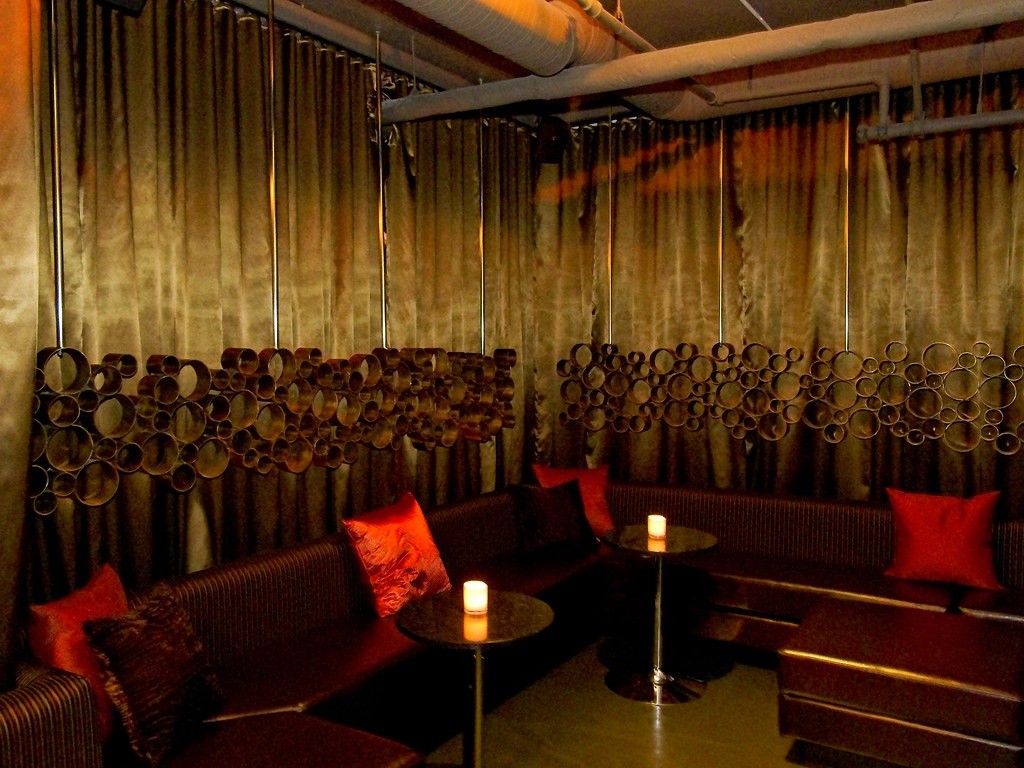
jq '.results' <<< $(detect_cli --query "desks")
[396,591,555,768]
[601,526,721,704]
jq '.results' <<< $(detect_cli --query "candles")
[464,615,488,642]
[647,537,665,551]
[648,515,666,537]
[463,581,487,614]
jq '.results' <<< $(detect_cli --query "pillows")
[31,564,130,741]
[515,480,599,559]
[885,486,1001,592]
[340,492,451,619]
[82,580,226,764]
[532,464,614,537]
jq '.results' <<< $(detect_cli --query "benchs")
[777,597,1024,768]
[0,484,599,768]
[600,483,1023,672]
